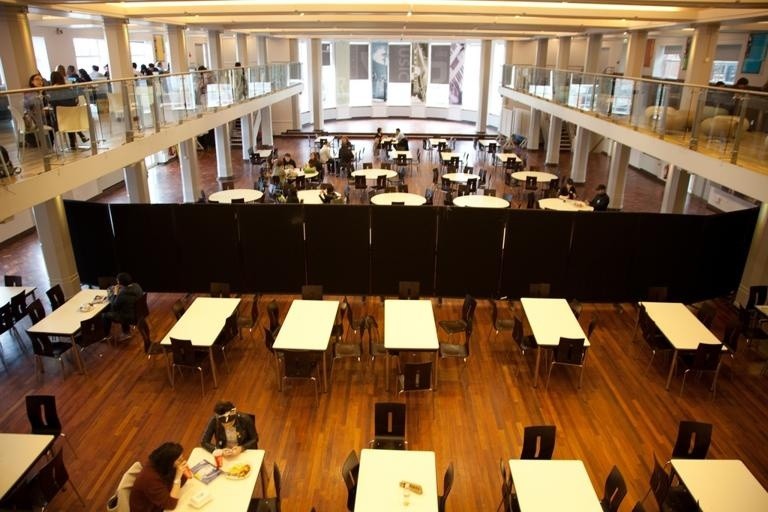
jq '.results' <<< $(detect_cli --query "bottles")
[397,481,422,494]
[402,492,410,506]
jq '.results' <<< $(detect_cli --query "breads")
[400,480,423,494]
[228,464,250,476]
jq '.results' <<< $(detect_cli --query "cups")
[212,449,224,468]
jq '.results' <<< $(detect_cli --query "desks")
[353,447,438,512]
[0,429,55,502]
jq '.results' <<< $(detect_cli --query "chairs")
[0,61,179,185]
[263,286,356,410]
[0,272,39,357]
[25,276,134,382]
[437,461,455,511]
[145,281,257,399]
[367,402,409,449]
[498,422,767,512]
[109,410,281,510]
[625,288,740,403]
[341,449,359,508]
[194,132,596,211]
[367,284,476,411]
[489,280,600,402]
[26,396,78,495]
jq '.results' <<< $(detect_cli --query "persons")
[372,128,403,155]
[21,58,245,153]
[128,442,192,512]
[557,178,575,199]
[266,134,352,205]
[714,78,748,126]
[585,184,608,211]
[199,401,258,457]
[98,272,144,343]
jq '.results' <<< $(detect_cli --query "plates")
[79,303,95,312]
[227,462,251,479]
